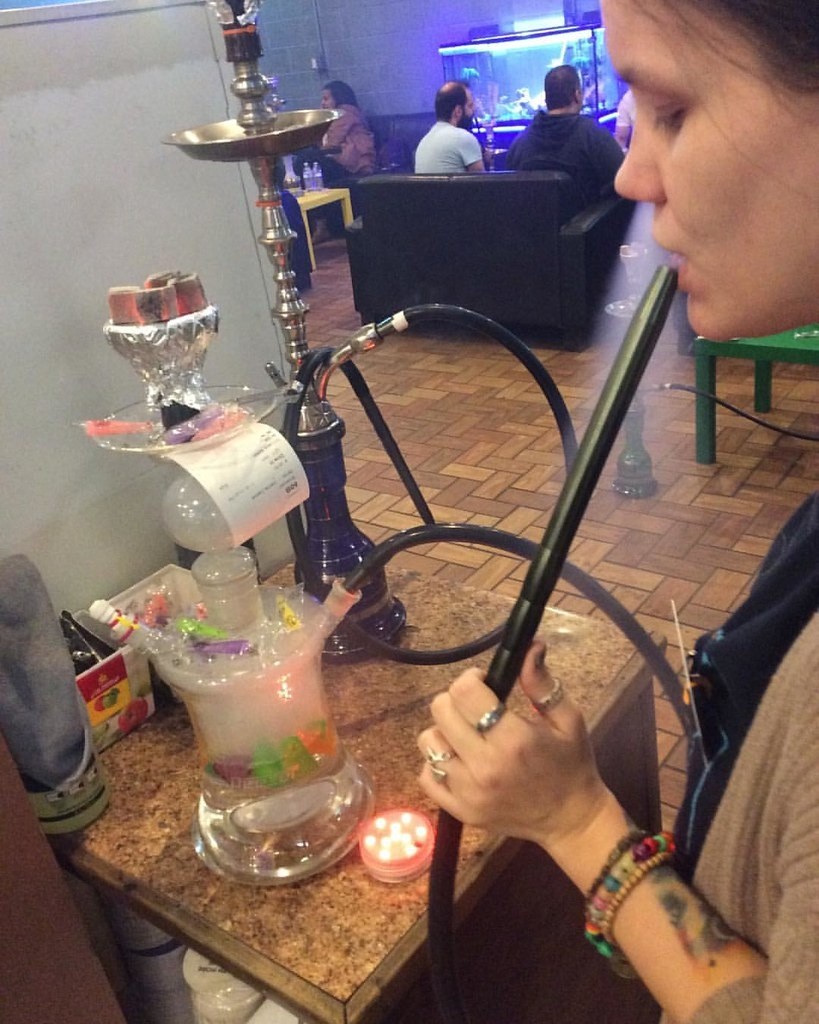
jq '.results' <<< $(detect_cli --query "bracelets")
[578,825,683,957]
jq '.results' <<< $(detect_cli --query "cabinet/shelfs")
[46,553,676,1024]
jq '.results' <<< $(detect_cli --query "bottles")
[313,162,324,191]
[303,162,314,192]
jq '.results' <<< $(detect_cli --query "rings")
[423,746,457,784]
[475,703,507,732]
[529,678,565,712]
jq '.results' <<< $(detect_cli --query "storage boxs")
[437,23,624,132]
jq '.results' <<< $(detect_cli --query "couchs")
[344,170,643,349]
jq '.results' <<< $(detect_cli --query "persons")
[614,82,641,152]
[276,160,314,296]
[505,66,626,202]
[295,80,377,244]
[416,0,819,1024]
[416,82,487,174]
